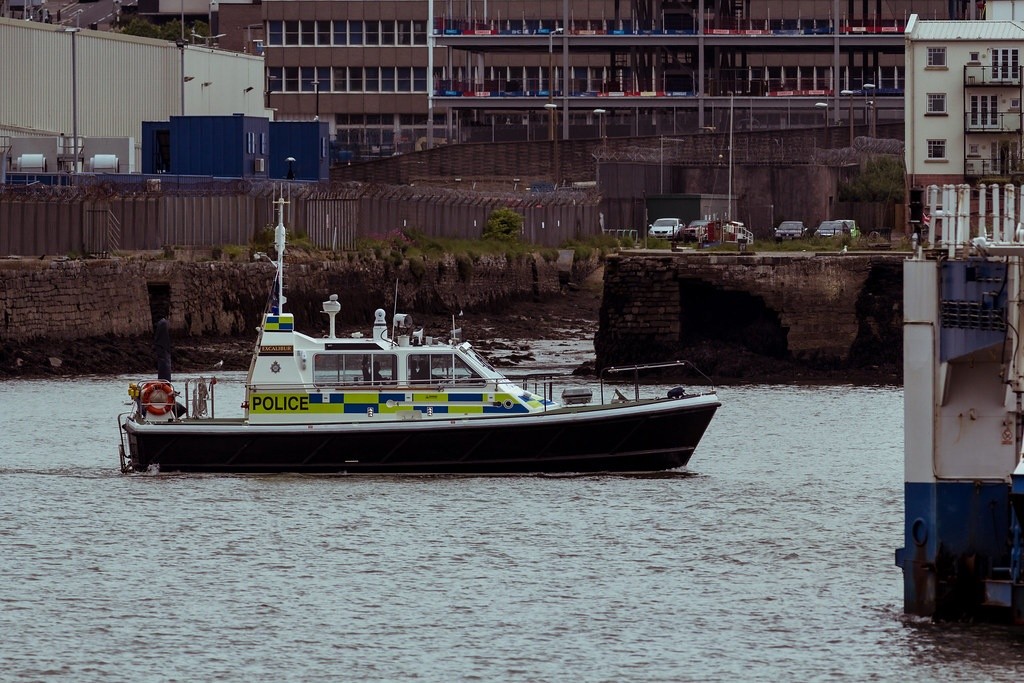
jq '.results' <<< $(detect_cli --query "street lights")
[548,27,565,98]
[842,89,855,162]
[815,102,829,126]
[864,83,876,125]
[65,26,83,173]
[594,108,607,136]
[544,104,558,141]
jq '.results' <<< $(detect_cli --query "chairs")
[967,164,975,175]
[361,357,370,385]
[411,360,417,384]
[982,163,989,175]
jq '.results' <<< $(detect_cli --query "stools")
[968,76,975,85]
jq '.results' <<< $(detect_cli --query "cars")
[835,219,861,238]
[815,221,852,239]
[647,217,687,241]
[773,220,809,243]
[683,219,718,243]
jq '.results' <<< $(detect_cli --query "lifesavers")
[141,383,174,416]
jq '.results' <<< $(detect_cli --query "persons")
[411,360,428,384]
[374,361,387,385]
[841,222,851,247]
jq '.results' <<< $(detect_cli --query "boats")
[129,181,722,476]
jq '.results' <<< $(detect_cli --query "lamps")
[244,86,253,92]
[184,76,195,82]
[202,82,212,86]
